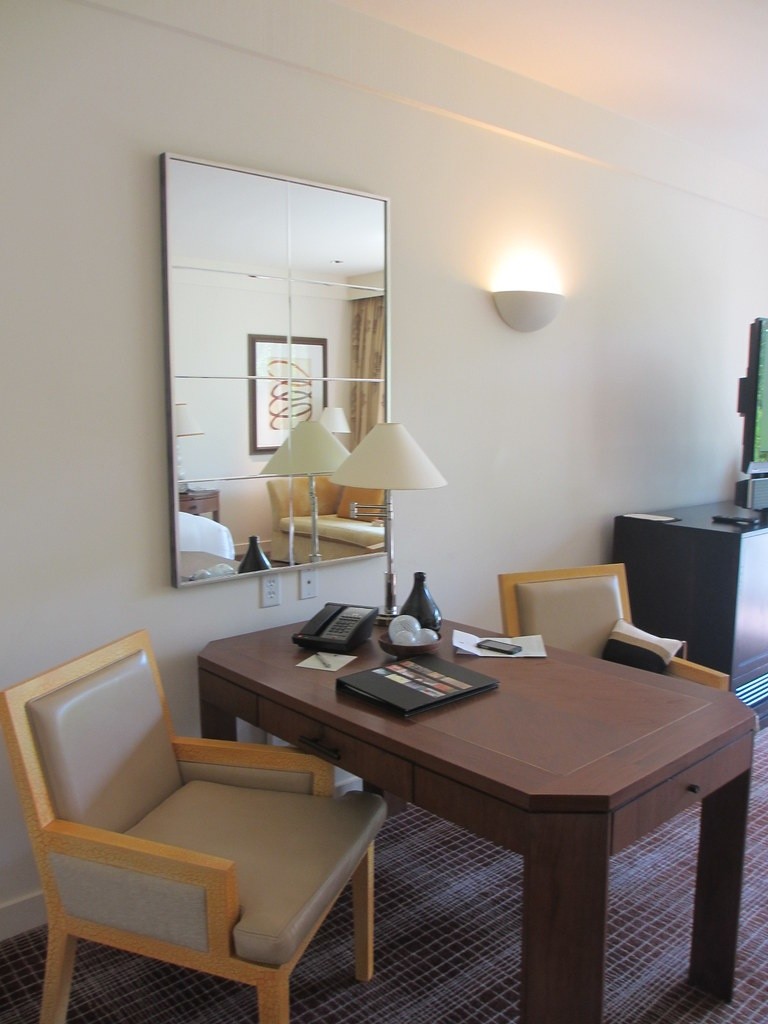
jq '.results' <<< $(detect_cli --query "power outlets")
[261,574,282,607]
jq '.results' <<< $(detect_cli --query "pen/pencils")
[310,649,333,667]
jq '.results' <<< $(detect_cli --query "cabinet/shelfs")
[179,490,221,522]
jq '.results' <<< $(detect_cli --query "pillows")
[601,619,684,673]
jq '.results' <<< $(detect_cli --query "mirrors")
[161,152,389,588]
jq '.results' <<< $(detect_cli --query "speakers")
[734,478,768,510]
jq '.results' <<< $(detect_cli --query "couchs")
[265,477,385,563]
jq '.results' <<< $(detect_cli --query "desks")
[180,551,241,581]
[198,618,758,1024]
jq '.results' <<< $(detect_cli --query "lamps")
[329,423,448,625]
[492,290,564,333]
[319,407,351,433]
[176,403,205,493]
[259,420,351,562]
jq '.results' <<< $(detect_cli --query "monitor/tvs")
[741,318,768,474]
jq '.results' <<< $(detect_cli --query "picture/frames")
[249,334,328,454]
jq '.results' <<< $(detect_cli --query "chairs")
[0,629,389,1024]
[497,564,730,691]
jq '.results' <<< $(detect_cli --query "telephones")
[292,601,380,655]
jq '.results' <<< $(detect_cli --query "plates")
[378,628,443,657]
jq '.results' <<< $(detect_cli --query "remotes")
[712,515,760,525]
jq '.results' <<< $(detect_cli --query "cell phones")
[477,639,522,655]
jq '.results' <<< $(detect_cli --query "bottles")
[397,571,442,633]
[237,535,272,575]
